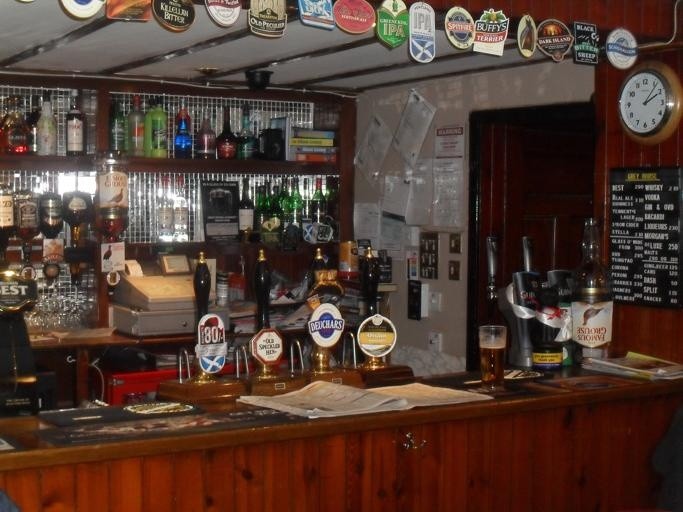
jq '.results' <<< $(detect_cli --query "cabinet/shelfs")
[1,69,356,407]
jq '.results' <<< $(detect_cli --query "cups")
[479,324,505,389]
[23,292,96,333]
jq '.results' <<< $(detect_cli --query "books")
[234,379,496,420]
[580,350,683,380]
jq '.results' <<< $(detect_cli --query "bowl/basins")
[245,70,273,90]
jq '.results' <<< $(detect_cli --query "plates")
[502,369,544,380]
[124,401,193,416]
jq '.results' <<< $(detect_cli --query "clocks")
[616,61,683,146]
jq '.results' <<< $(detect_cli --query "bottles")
[215,271,229,306]
[95,152,129,243]
[64,88,85,157]
[192,252,211,325]
[108,94,270,159]
[531,285,564,369]
[156,176,173,242]
[311,247,325,284]
[12,190,40,266]
[25,121,36,154]
[573,218,613,364]
[39,193,62,264]
[0,184,12,267]
[36,95,57,157]
[0,95,28,155]
[173,176,188,243]
[254,249,270,322]
[238,175,337,249]
[360,246,375,294]
[62,191,91,251]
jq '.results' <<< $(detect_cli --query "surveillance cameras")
[247,71,271,92]
